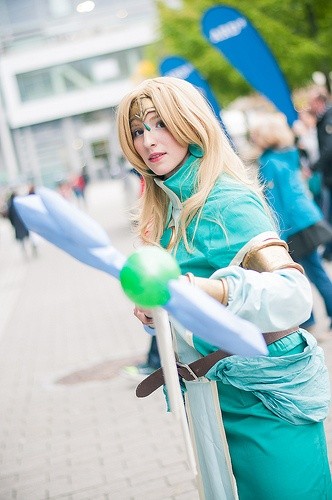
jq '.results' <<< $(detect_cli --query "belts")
[136,325,299,398]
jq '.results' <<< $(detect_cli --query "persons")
[59,165,90,205]
[249,111,331,343]
[6,181,39,262]
[116,77,332,500]
[293,85,332,265]
